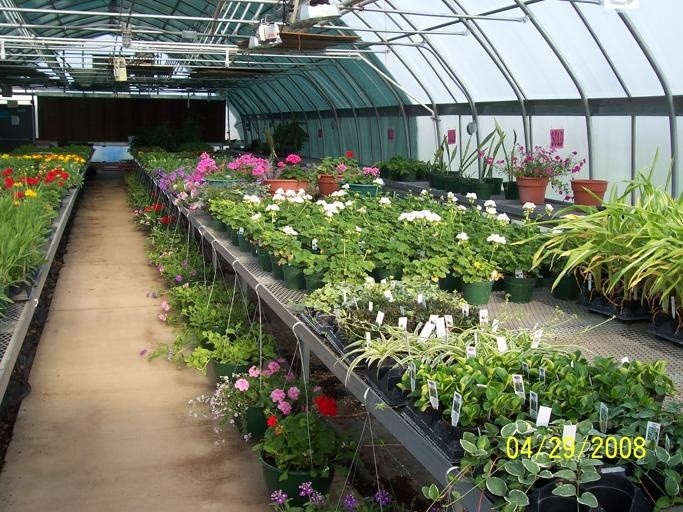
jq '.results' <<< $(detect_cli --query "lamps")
[122,23,131,48]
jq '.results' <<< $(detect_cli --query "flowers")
[122,138,683,512]
[1,140,94,323]
